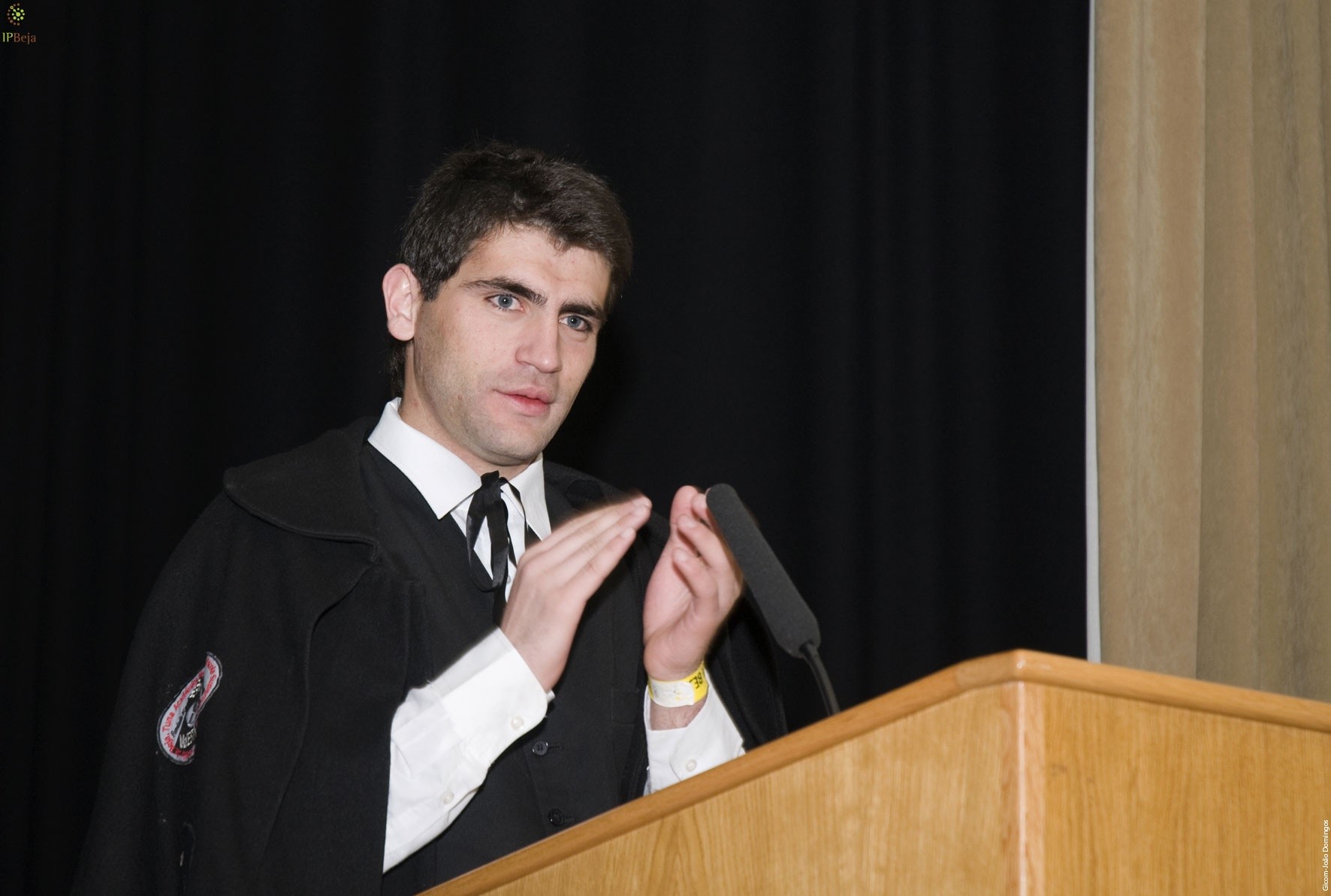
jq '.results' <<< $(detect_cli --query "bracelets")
[647,659,709,708]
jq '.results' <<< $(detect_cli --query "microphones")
[705,482,839,715]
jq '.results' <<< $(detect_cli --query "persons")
[81,142,788,896]
